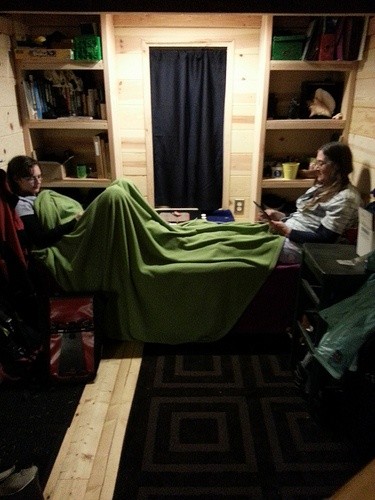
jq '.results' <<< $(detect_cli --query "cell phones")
[253,201,271,221]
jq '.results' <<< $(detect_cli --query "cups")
[282,162,300,179]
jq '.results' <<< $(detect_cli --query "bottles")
[271,166,283,178]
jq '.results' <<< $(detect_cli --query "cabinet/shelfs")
[9,6,118,190]
[251,16,370,224]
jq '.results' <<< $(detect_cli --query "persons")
[179,142,359,266]
[7,155,141,268]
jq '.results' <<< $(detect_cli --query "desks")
[300,241,364,333]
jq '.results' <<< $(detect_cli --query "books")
[24,74,106,119]
[94,135,112,179]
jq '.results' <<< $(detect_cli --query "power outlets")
[233,199,244,214]
